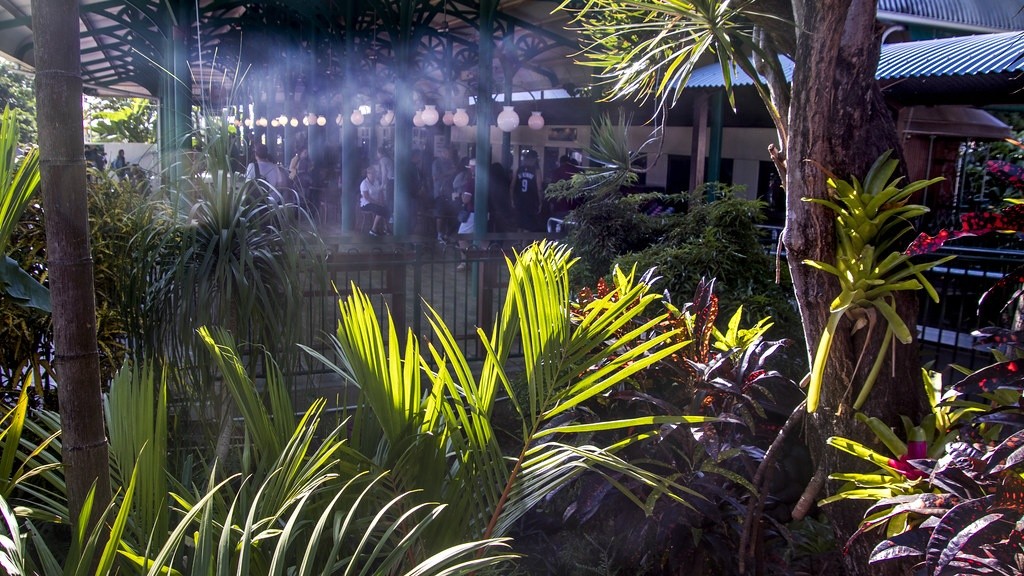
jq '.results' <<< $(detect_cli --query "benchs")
[448,232,570,241]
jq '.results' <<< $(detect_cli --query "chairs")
[316,188,338,226]
[548,218,576,233]
[356,192,393,236]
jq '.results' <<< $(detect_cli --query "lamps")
[227,81,545,132]
[562,83,580,98]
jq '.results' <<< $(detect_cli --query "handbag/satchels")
[245,162,269,202]
[458,210,469,222]
[111,157,118,169]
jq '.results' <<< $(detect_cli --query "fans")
[421,0,475,39]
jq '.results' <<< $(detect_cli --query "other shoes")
[437,238,447,243]
[457,263,466,270]
[368,229,377,236]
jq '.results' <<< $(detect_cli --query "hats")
[465,158,475,168]
[525,150,539,157]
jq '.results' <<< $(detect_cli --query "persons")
[86,144,130,184]
[244,135,575,273]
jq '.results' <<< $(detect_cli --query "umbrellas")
[895,102,1011,236]
